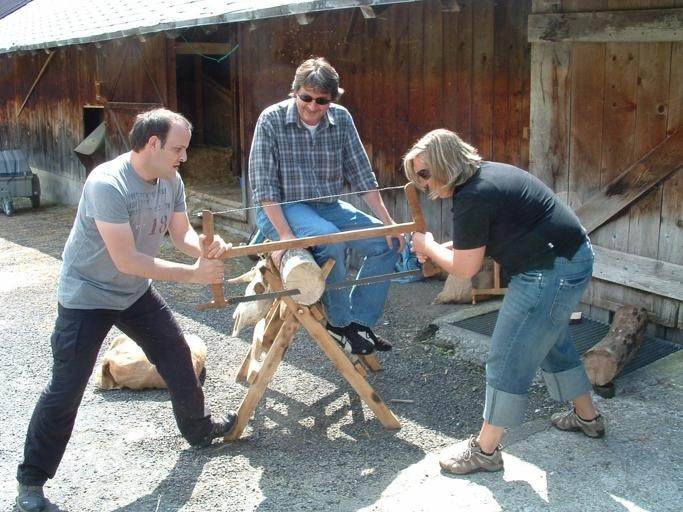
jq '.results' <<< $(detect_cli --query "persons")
[246,54,407,360]
[402,126,605,476]
[12,105,242,511]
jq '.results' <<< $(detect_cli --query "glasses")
[417,168,433,180]
[298,93,330,105]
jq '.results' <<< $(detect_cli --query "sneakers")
[191,409,239,450]
[438,436,503,475]
[18,485,45,511]
[551,409,603,438]
[325,322,392,355]
